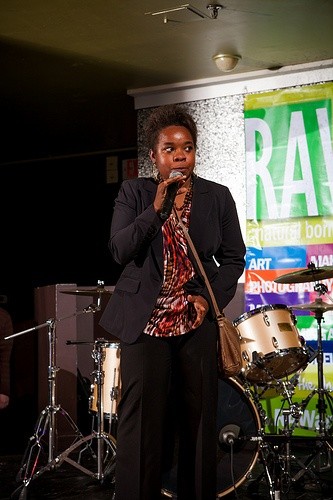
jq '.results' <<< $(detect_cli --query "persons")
[98,104,246,500]
[0,307,14,409]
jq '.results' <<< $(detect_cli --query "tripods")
[4,297,118,500]
[249,313,333,500]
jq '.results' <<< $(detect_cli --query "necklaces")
[156,174,193,227]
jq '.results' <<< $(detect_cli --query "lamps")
[212,53,241,72]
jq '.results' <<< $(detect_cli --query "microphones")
[222,431,235,444]
[160,171,182,219]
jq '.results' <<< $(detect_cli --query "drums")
[85,338,122,419]
[161,375,264,499]
[229,304,309,382]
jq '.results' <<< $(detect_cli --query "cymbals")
[289,302,333,312]
[274,265,333,284]
[59,286,114,295]
